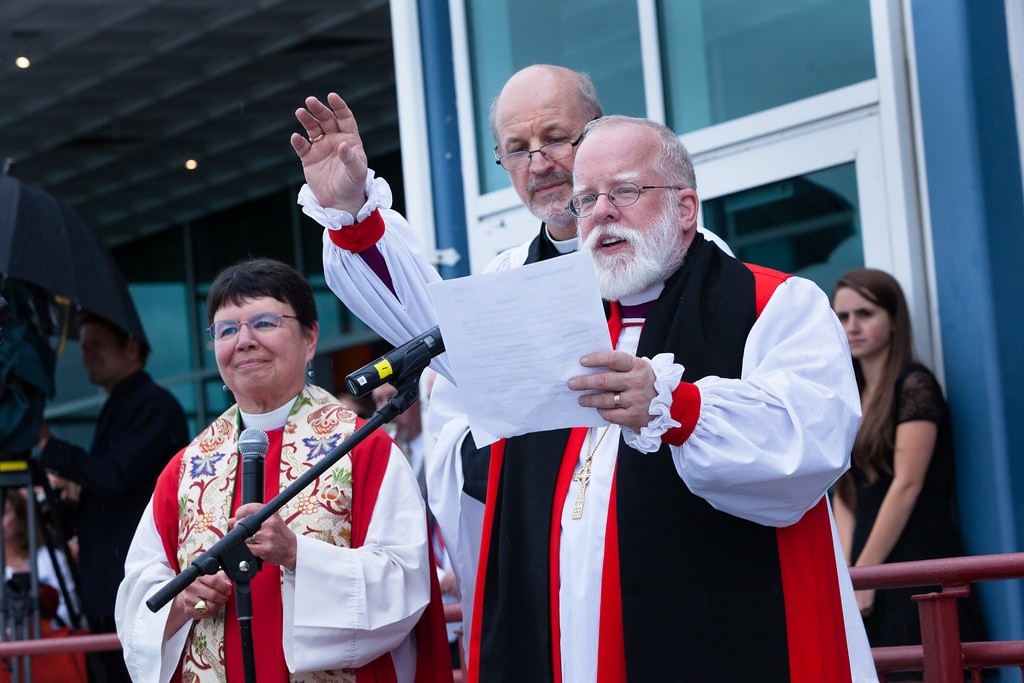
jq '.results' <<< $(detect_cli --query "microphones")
[238,428,270,505]
[347,325,447,397]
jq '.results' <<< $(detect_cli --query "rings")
[250,534,256,544]
[308,133,325,144]
[614,391,621,409]
[194,599,208,615]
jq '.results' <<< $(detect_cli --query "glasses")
[206,312,297,341]
[565,182,682,221]
[494,117,599,170]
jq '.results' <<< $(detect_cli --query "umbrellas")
[0,172,147,342]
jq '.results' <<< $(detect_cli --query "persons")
[291,64,878,683]
[0,314,191,683]
[830,268,957,683]
[114,260,453,683]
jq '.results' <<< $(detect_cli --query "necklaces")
[573,426,610,519]
